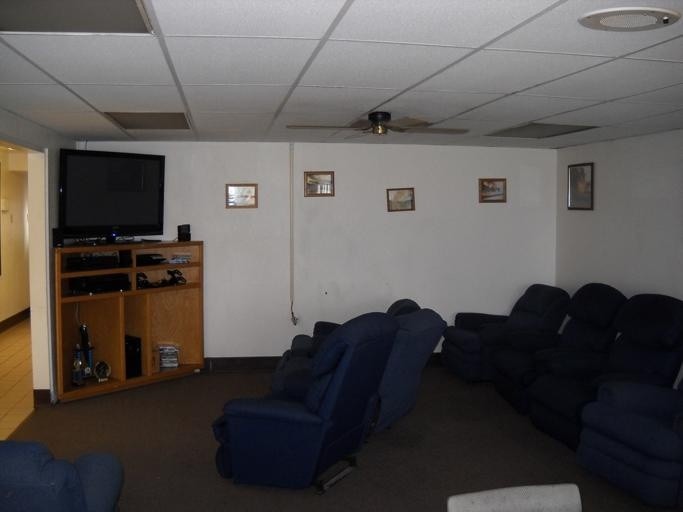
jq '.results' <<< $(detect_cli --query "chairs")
[477,281,629,417]
[209,310,398,493]
[438,284,569,383]
[271,297,448,434]
[271,311,398,441]
[289,298,421,350]
[525,291,682,451]
[574,373,682,511]
[0,438,125,511]
[444,481,583,511]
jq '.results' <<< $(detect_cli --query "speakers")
[53,228,64,247]
[178,224,191,241]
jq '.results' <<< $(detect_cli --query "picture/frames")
[478,178,506,203]
[303,171,335,197]
[224,184,258,209]
[566,162,593,210]
[385,188,415,212]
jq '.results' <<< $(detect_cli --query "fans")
[286,109,469,143]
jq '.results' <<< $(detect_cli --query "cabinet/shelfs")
[52,239,207,404]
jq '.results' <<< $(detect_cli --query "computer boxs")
[125,335,141,379]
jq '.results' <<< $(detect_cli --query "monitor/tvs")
[59,148,165,244]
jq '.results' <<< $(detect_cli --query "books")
[155,344,179,367]
